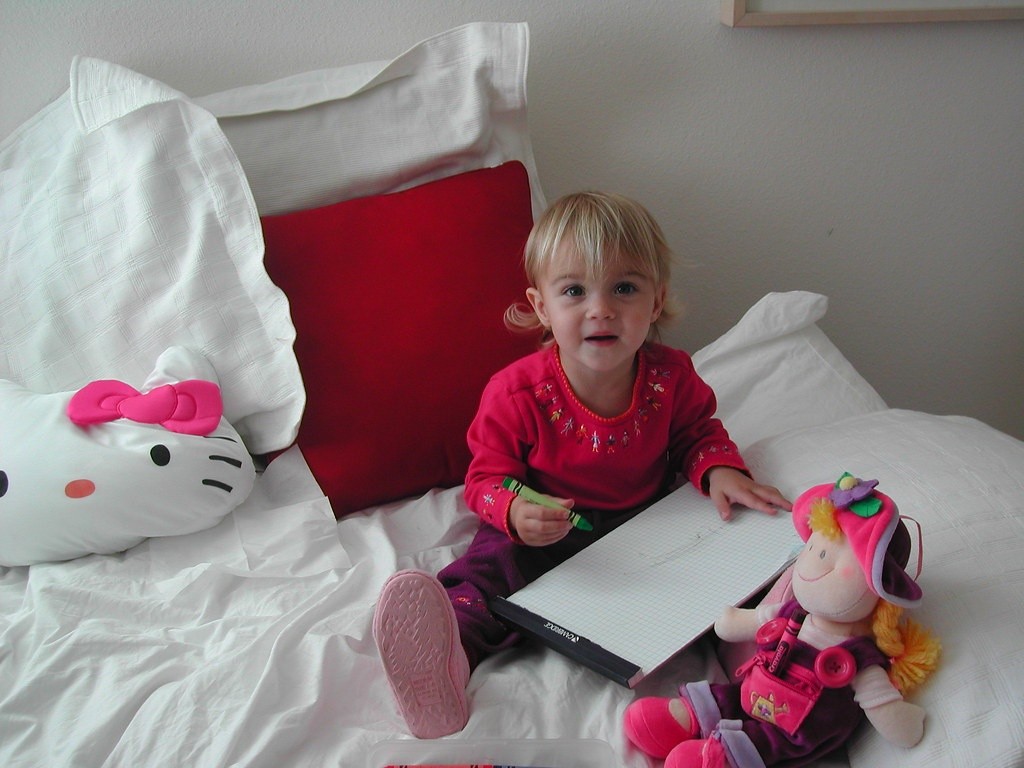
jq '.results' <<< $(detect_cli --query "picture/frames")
[722,1,1024,27]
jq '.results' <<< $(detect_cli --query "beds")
[0,485,848,768]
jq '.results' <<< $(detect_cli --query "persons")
[370,191,794,740]
[625,471,940,768]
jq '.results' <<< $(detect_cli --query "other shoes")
[755,561,794,610]
[369,566,469,739]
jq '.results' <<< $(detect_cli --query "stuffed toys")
[0,345,255,567]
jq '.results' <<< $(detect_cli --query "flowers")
[830,471,884,518]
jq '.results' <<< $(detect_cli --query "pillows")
[743,407,1024,768]
[193,22,549,229]
[692,291,890,458]
[1,52,305,454]
[257,160,550,520]
